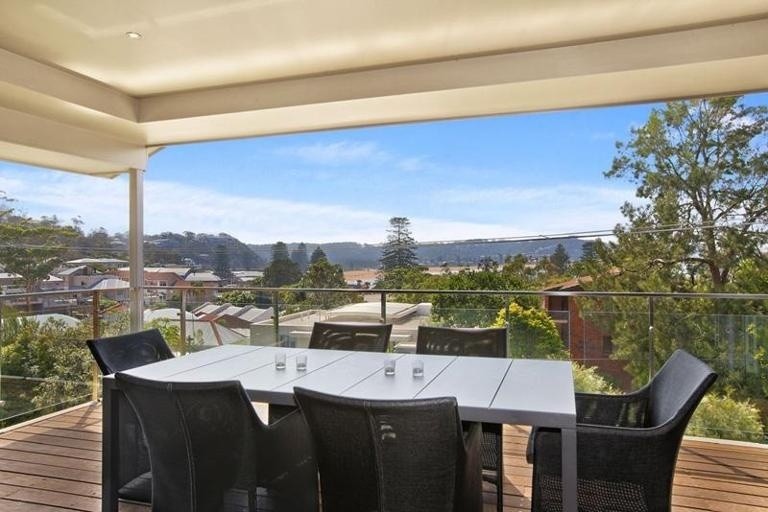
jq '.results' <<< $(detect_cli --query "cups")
[296,355,307,370]
[383,358,396,375]
[412,359,425,377]
[275,352,286,369]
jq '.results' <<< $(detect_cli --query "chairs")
[86,329,178,512]
[409,319,513,512]
[265,321,394,426]
[520,343,721,511]
[287,383,489,512]
[116,367,322,512]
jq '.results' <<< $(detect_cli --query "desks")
[92,340,582,511]
